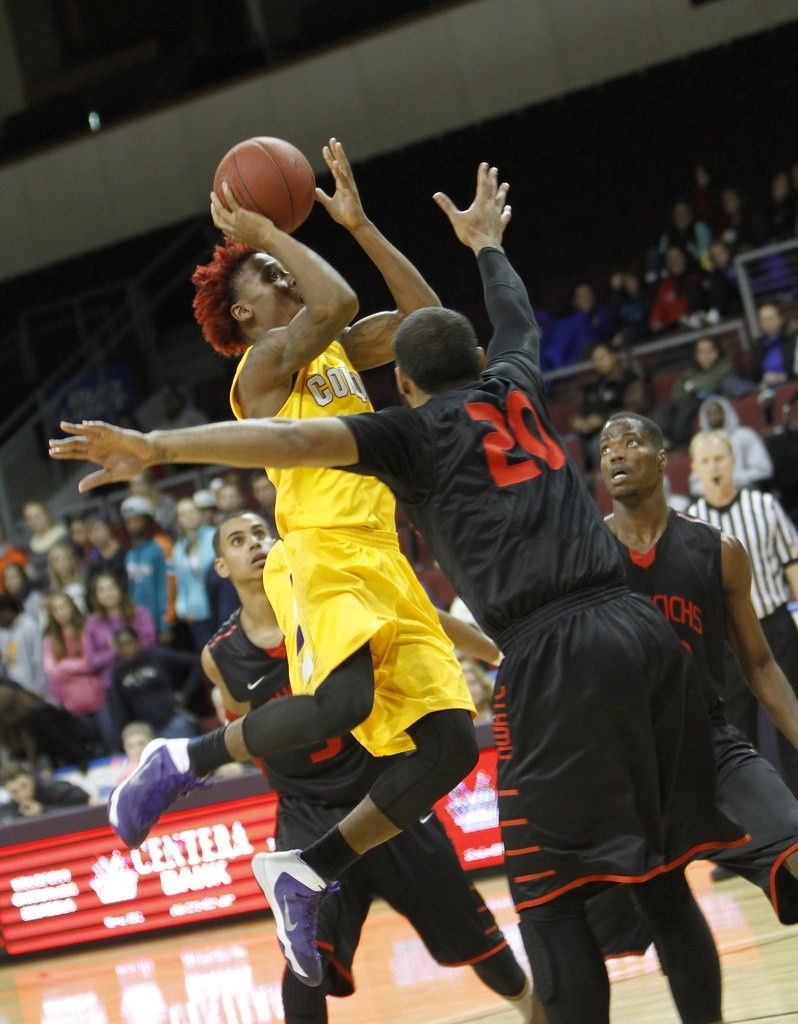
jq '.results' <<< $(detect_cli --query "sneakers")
[106,736,213,851]
[251,847,342,986]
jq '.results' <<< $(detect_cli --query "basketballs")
[212,134,319,243]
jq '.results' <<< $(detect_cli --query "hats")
[194,490,217,509]
[121,496,155,519]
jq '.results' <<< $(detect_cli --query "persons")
[203,509,544,1024]
[531,410,797,1019]
[48,162,797,1022]
[0,164,798,826]
[108,137,478,987]
[685,431,797,882]
